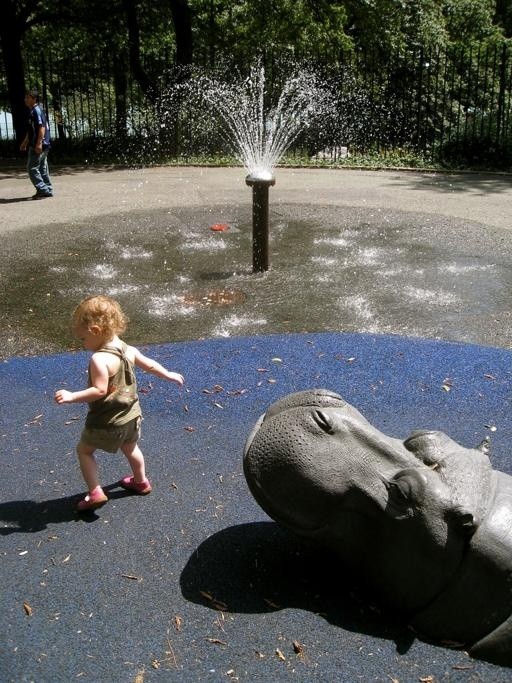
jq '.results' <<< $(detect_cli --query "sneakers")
[121,475,152,496]
[77,487,109,511]
[40,193,52,197]
[32,189,49,200]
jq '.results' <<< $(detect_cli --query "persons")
[54,294,185,511]
[20,93,53,200]
